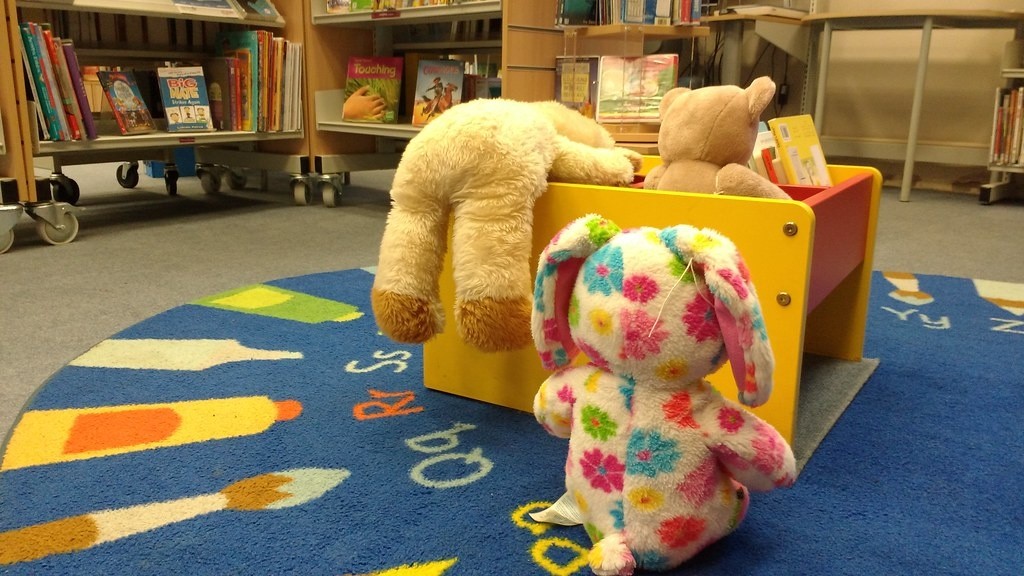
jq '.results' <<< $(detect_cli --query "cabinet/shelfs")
[556,5,815,155]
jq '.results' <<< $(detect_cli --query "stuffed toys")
[529,214,797,576]
[371,96,642,355]
[644,77,793,201]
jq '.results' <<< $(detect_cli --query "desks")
[801,9,1024,202]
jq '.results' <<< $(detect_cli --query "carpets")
[0,264,1024,576]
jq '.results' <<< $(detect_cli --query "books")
[993,86,1024,166]
[748,114,832,187]
[16,0,503,141]
[554,0,703,26]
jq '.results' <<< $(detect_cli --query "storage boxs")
[145,146,198,178]
[423,155,884,456]
